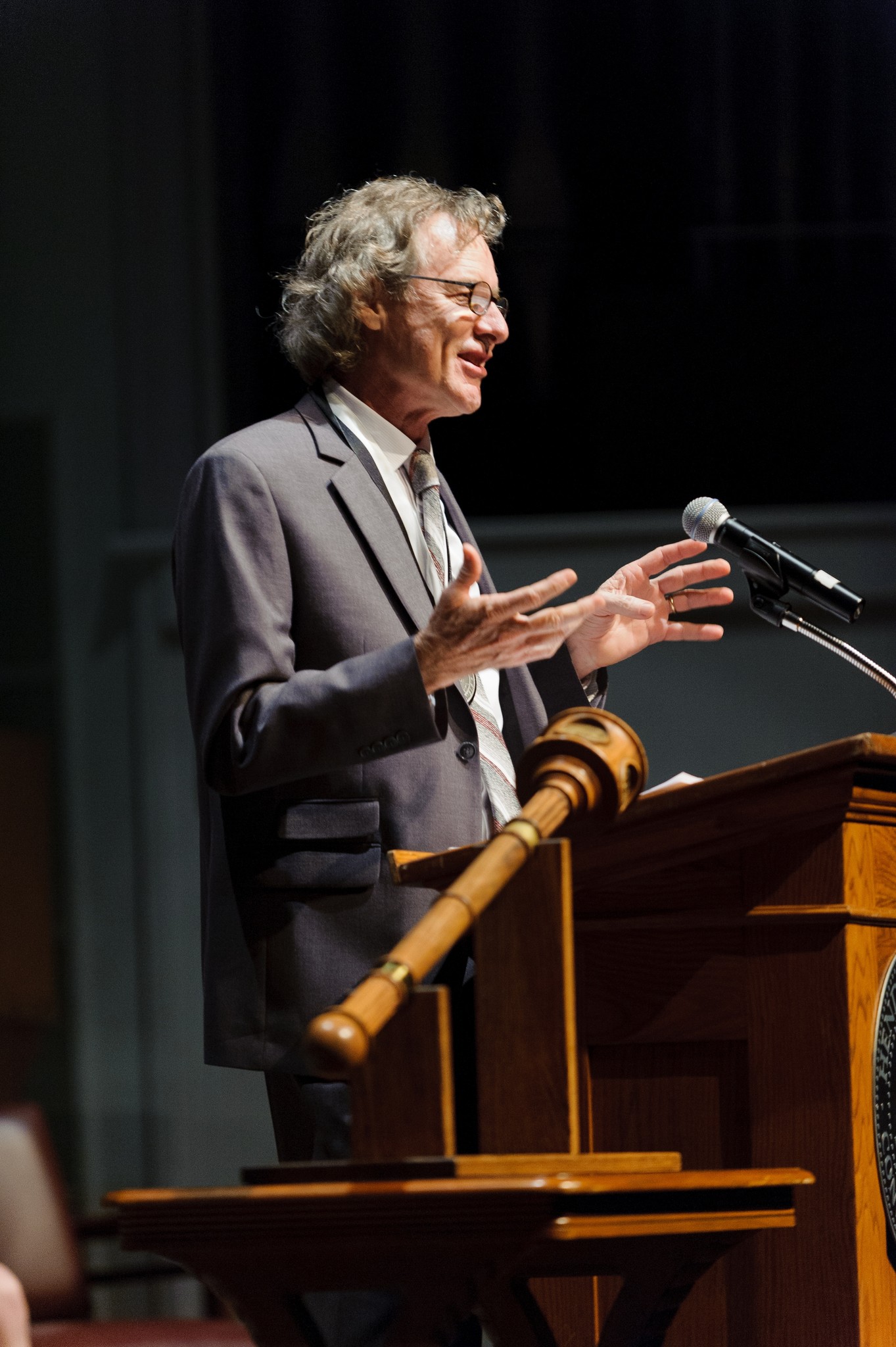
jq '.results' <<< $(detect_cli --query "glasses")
[386,272,509,317]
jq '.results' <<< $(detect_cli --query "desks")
[109,1155,818,1346]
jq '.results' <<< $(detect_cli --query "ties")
[412,453,488,713]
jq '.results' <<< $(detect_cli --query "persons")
[177,174,734,1149]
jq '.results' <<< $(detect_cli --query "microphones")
[682,495,867,625]
